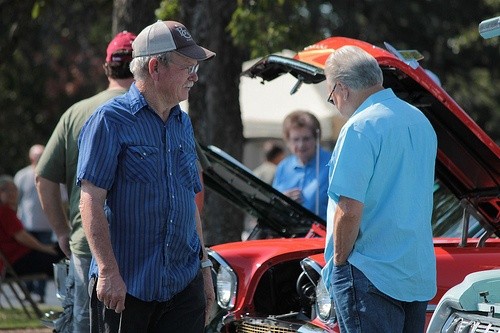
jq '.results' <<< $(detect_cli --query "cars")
[45,16,500,333]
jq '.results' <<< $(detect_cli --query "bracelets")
[201,260,213,268]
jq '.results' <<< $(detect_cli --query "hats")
[106,31,137,63]
[132,19,216,63]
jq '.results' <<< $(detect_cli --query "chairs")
[0,249,48,320]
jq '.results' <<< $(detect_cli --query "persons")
[244,140,285,233]
[35,30,137,333]
[272,111,333,223]
[14,145,69,294]
[0,176,58,282]
[320,45,438,333]
[77,19,216,333]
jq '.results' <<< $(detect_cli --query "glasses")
[288,136,315,143]
[166,59,200,75]
[327,83,337,105]
